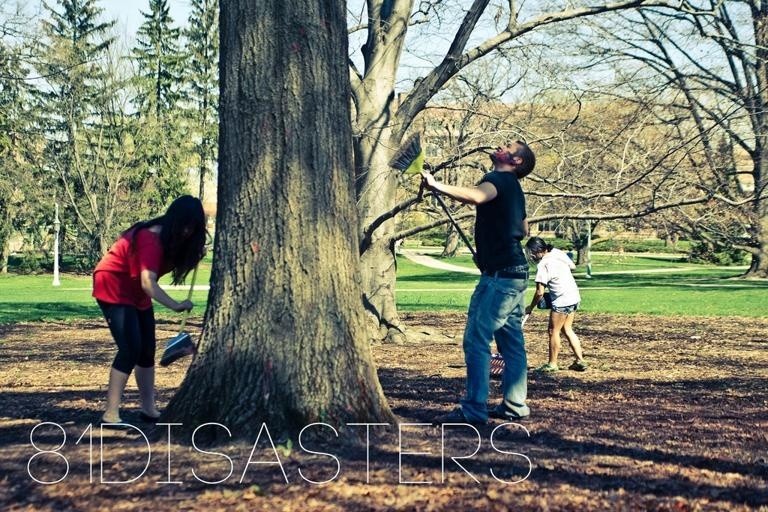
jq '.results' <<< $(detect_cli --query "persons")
[90,193,214,431]
[524,236,582,372]
[424,139,537,428]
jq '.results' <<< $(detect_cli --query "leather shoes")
[138,410,162,422]
[96,418,131,430]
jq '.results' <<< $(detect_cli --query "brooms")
[158,262,201,366]
[490,314,547,379]
[388,131,478,270]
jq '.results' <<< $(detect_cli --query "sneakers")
[569,361,587,371]
[488,408,529,421]
[432,407,489,426]
[533,364,559,373]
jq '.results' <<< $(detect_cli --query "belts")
[486,270,529,279]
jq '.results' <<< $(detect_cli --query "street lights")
[51,194,61,286]
